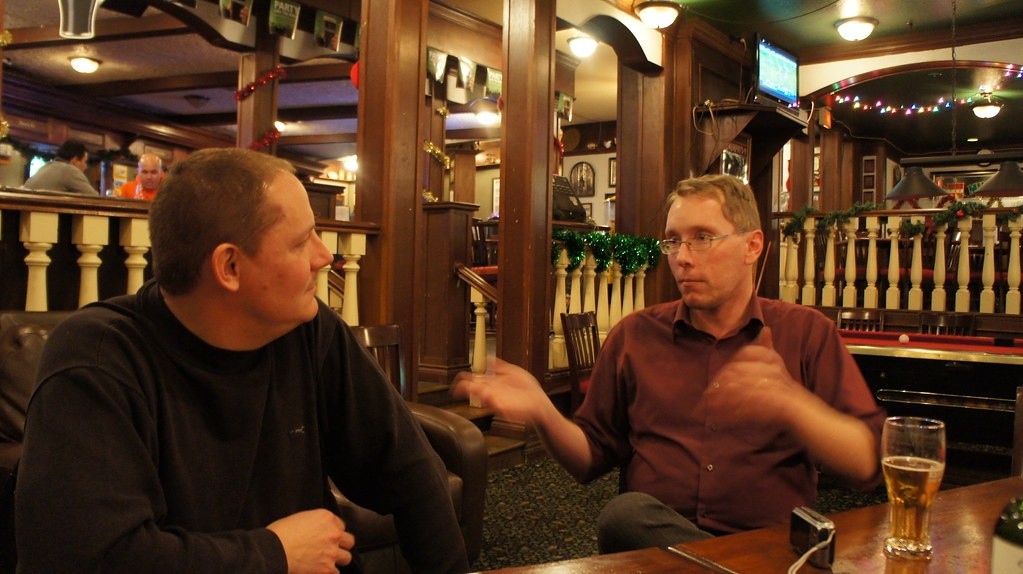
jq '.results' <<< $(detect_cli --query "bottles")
[134,182,143,199]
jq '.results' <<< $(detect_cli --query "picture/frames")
[609,158,616,187]
[582,203,592,218]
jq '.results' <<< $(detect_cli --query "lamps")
[883,166,948,199]
[974,160,1023,197]
[566,36,599,60]
[969,99,1004,118]
[634,2,683,30]
[832,16,879,42]
[68,43,104,74]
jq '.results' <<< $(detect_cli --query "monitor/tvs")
[753,32,800,109]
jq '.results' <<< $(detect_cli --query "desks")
[470,477,1023,574]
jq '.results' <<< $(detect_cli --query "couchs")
[0,311,487,574]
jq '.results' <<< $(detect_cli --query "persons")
[457,170,881,556]
[115,153,168,202]
[14,148,460,574]
[22,139,99,195]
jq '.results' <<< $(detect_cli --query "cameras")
[790,506,835,569]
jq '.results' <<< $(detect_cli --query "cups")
[106,189,121,197]
[882,418,949,562]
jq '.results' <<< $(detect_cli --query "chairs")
[918,311,976,336]
[560,311,600,400]
[348,325,406,400]
[836,308,887,332]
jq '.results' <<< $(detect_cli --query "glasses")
[655,228,746,255]
